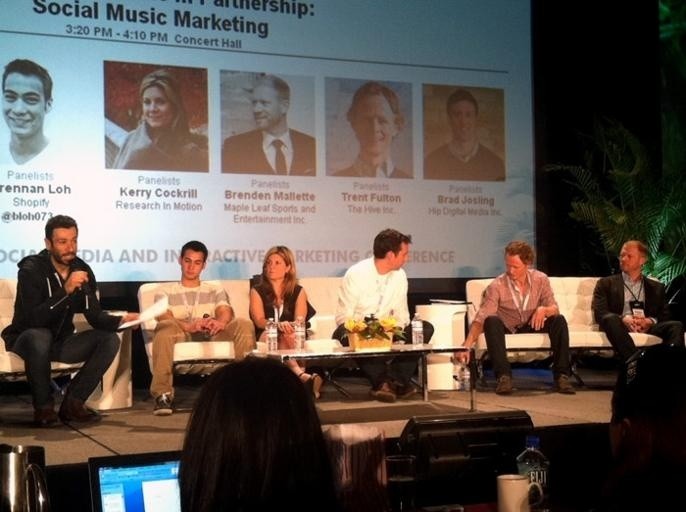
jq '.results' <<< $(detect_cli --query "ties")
[271,140,287,176]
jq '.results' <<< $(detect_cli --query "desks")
[262,337,466,404]
[70,308,143,411]
[411,300,470,394]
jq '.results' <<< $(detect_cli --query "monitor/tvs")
[87,449,187,512]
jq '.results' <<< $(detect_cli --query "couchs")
[135,278,423,405]
[466,276,664,388]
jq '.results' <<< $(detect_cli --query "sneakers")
[369,380,396,403]
[59,397,101,424]
[394,380,418,399]
[34,400,64,428]
[552,373,576,394]
[153,392,173,415]
[495,374,513,395]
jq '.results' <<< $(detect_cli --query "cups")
[385,454,418,511]
[0,443,50,512]
[496,473,544,512]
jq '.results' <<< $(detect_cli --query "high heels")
[298,372,322,401]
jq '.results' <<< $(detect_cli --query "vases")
[345,326,397,351]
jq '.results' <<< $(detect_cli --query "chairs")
[0,281,88,421]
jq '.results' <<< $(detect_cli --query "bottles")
[411,311,424,351]
[463,362,470,392]
[517,436,548,512]
[264,317,278,354]
[294,316,306,355]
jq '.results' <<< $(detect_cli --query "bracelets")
[293,314,306,323]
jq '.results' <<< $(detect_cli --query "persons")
[148,240,257,417]
[329,79,414,178]
[249,244,326,402]
[608,346,685,512]
[449,239,577,395]
[591,240,686,382]
[330,227,435,403]
[423,85,506,182]
[221,74,315,175]
[178,354,328,512]
[1,215,142,428]
[1,58,53,169]
[111,67,208,172]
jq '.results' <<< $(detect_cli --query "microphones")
[69,259,90,295]
[202,313,209,318]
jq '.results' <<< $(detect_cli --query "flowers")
[338,310,404,332]
